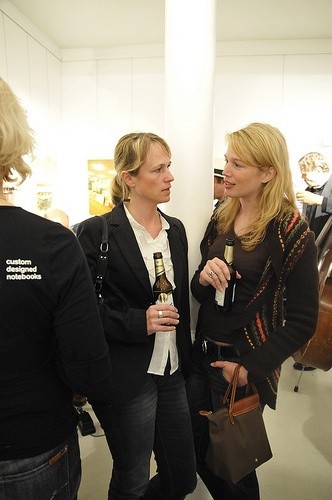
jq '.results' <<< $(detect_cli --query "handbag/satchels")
[94,214,109,324]
[199,365,272,483]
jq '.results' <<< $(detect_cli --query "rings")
[207,271,214,277]
[158,310,164,318]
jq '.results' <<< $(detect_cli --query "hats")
[214,157,227,177]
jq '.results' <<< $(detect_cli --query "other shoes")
[293,363,316,371]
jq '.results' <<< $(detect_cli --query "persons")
[73,131,199,500]
[0,76,114,500]
[188,122,320,500]
[292,151,331,372]
[214,158,228,213]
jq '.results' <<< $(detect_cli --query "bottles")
[151,252,177,330]
[214,239,236,314]
